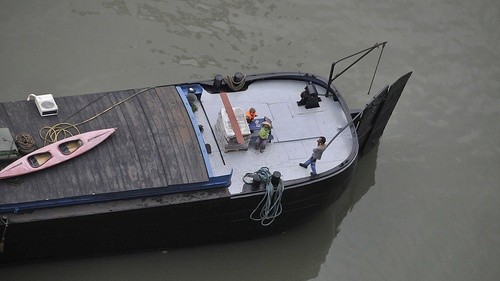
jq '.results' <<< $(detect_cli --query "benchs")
[250,135,273,141]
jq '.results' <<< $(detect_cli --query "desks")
[248,117,272,143]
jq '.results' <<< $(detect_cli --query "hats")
[262,121,271,128]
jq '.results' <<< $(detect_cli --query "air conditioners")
[27,93,59,116]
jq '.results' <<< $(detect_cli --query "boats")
[0,40,413,264]
[0,126,119,180]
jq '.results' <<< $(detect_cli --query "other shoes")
[299,163,307,168]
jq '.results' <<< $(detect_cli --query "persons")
[254,121,271,153]
[298,136,326,176]
[245,107,258,123]
